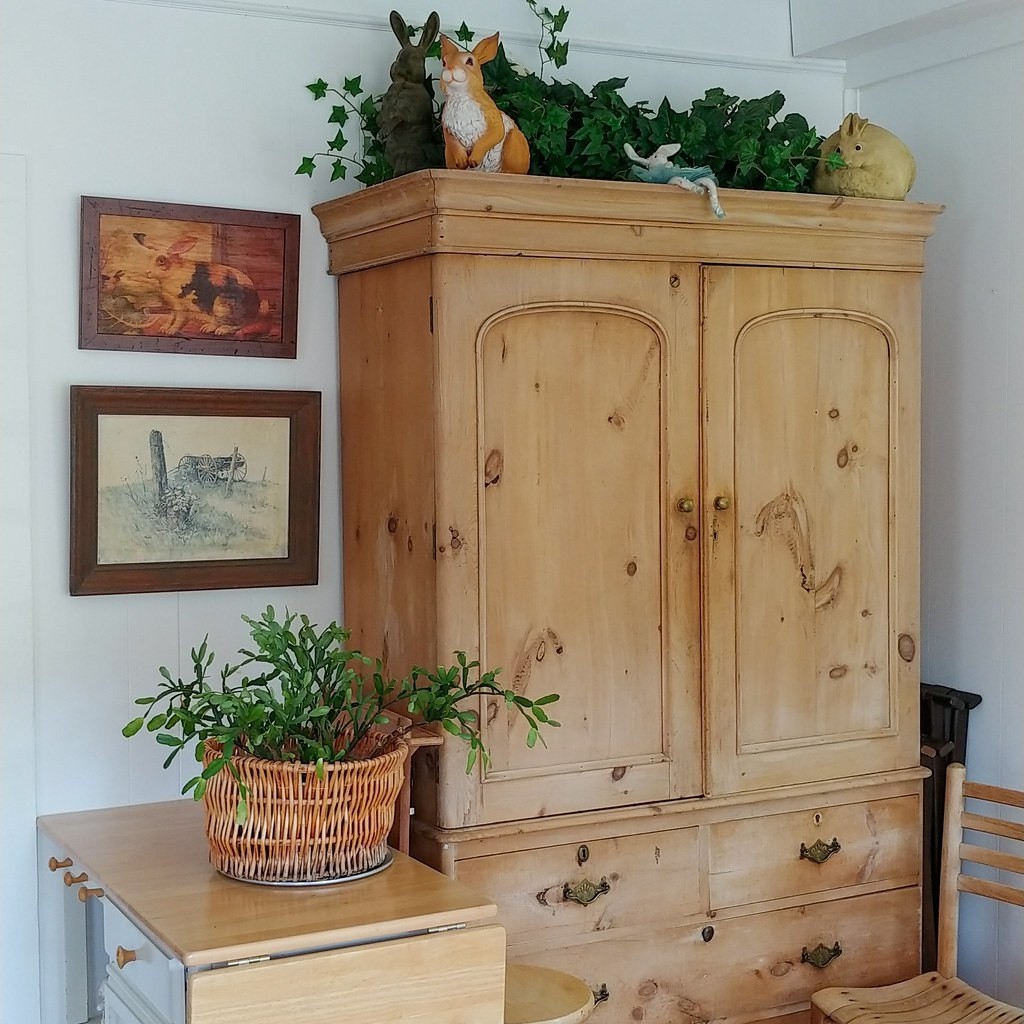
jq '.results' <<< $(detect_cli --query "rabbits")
[377,9,441,180]
[812,113,917,201]
[623,142,727,219]
[438,30,530,174]
[131,233,270,337]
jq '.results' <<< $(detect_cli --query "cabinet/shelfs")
[311,171,923,1023]
[35,806,510,1023]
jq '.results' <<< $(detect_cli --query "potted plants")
[123,603,561,882]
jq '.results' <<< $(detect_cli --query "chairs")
[809,764,1023,1024]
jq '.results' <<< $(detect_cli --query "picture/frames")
[70,383,321,598]
[80,193,302,358]
